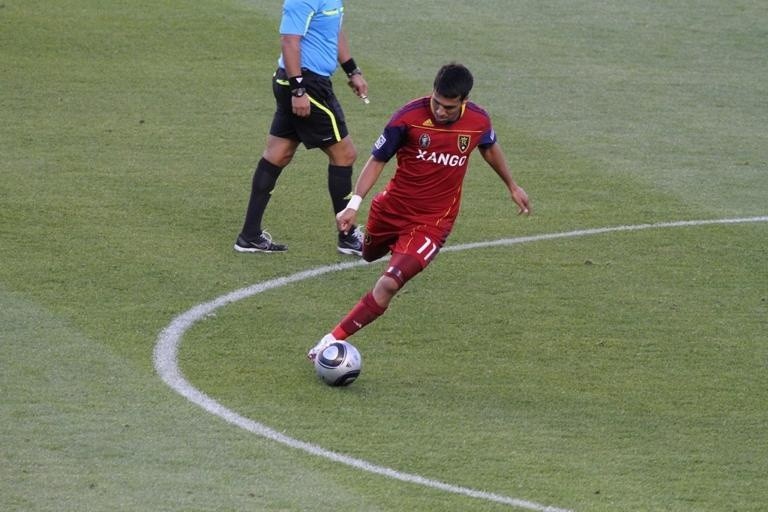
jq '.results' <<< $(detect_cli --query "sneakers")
[234,233,288,252]
[336,224,366,256]
[307,333,337,362]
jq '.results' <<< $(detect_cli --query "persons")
[234,0,369,256]
[308,64,531,363]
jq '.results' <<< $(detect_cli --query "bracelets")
[339,60,356,73]
[346,193,362,212]
[288,76,305,88]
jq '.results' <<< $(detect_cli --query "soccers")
[315,340,361,386]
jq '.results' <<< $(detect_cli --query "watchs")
[346,68,364,79]
[292,91,306,98]
